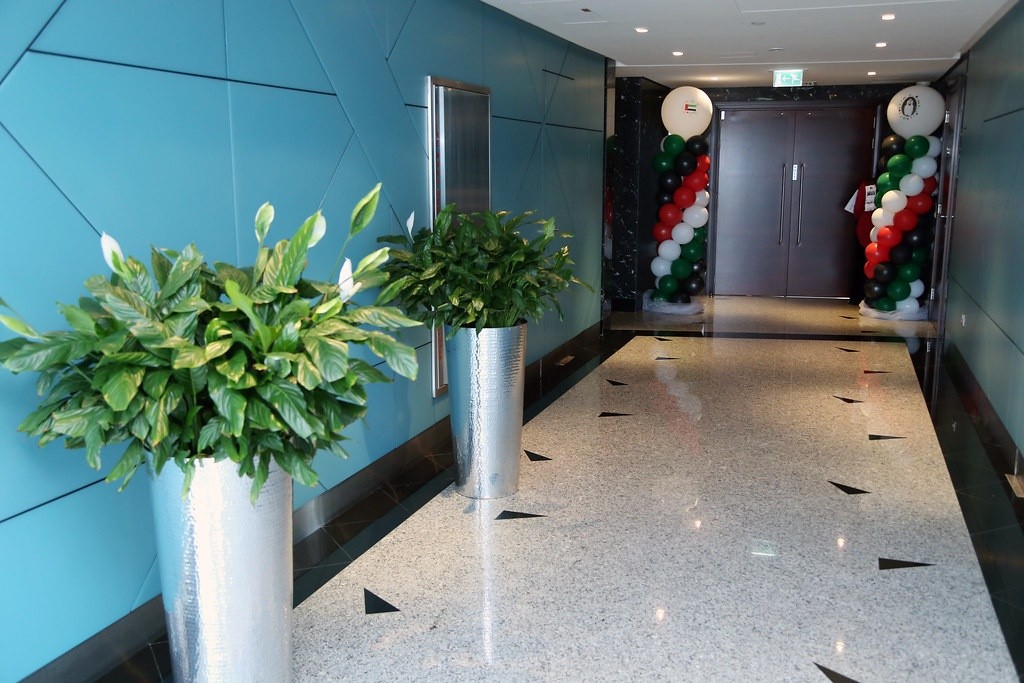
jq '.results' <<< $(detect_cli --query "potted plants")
[0,180,425,683]
[375,202,594,501]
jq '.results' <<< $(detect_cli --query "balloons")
[864,297,875,309]
[670,258,692,278]
[682,275,704,295]
[690,261,706,277]
[650,288,669,303]
[650,255,673,277]
[661,85,713,140]
[874,296,896,311]
[865,133,938,301]
[658,274,680,296]
[679,240,705,262]
[657,239,681,261]
[886,84,944,140]
[894,297,919,313]
[670,291,691,304]
[655,277,661,290]
[648,133,716,253]
